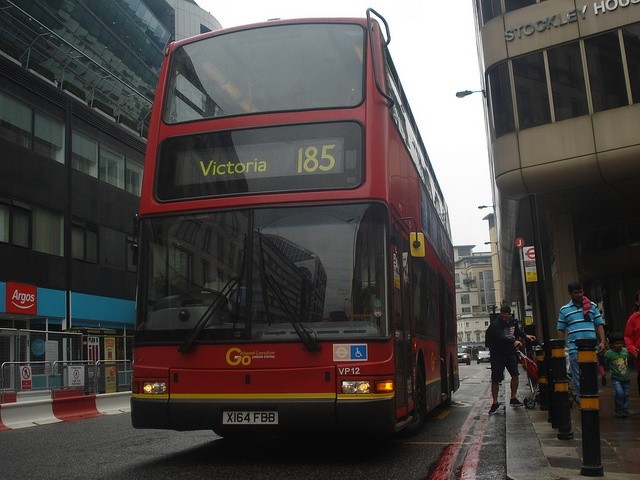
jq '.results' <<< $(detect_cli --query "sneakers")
[623,410,634,417]
[489,402,502,415]
[509,397,523,407]
[613,410,627,418]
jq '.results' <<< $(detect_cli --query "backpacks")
[485,318,520,350]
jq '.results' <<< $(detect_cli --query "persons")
[624,290,640,399]
[556,280,607,410]
[602,330,630,420]
[241,244,292,330]
[484,305,538,416]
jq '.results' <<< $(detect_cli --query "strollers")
[515,340,579,410]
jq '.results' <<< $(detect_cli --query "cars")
[476,350,490,364]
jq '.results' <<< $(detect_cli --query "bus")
[130,8,459,448]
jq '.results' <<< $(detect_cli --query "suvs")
[457,347,471,365]
